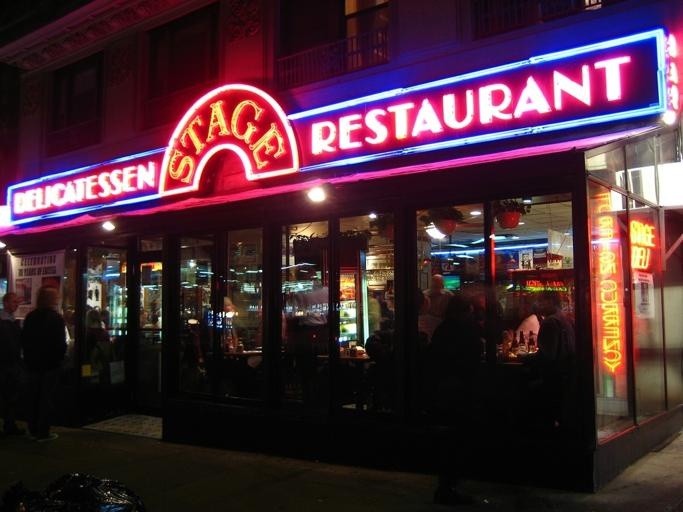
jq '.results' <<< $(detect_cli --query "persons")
[20,286,66,443]
[62,305,110,367]
[140,309,162,330]
[415,275,577,374]
[1,293,25,438]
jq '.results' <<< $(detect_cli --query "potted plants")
[493,198,531,228]
[369,212,394,240]
[419,206,469,234]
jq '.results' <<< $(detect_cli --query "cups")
[81,365,91,377]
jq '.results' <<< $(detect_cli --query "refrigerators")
[297,239,369,350]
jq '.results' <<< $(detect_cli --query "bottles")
[511,328,536,354]
[349,343,356,357]
